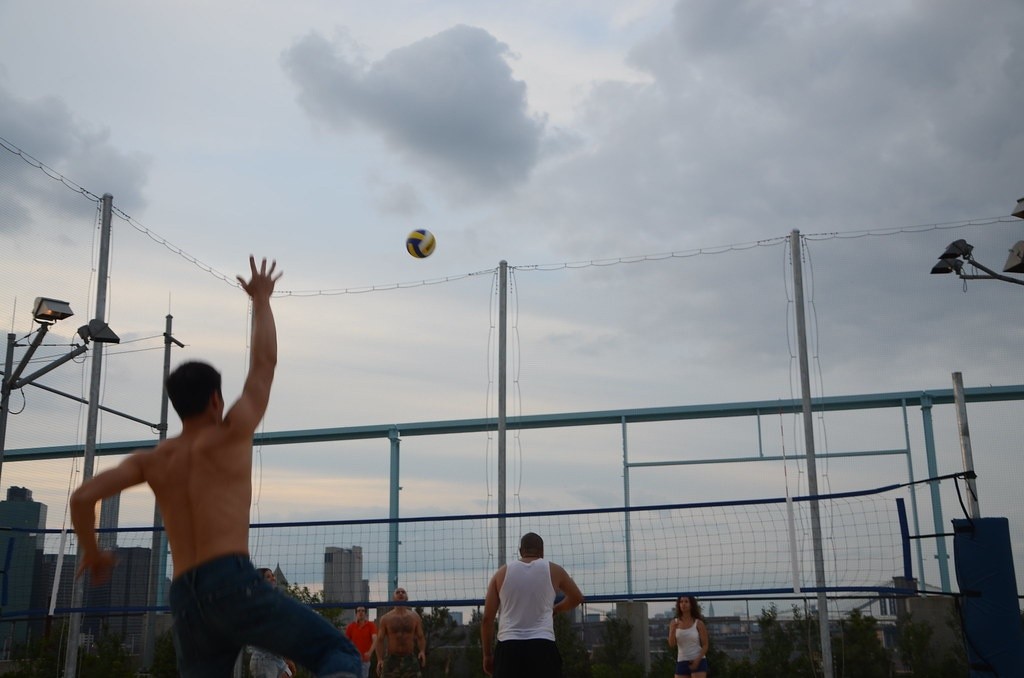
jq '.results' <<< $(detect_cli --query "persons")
[668,593,709,678]
[345,605,378,678]
[69,255,361,678]
[247,568,296,678]
[375,588,426,678]
[479,533,583,678]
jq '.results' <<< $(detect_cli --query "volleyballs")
[406,228,436,259]
